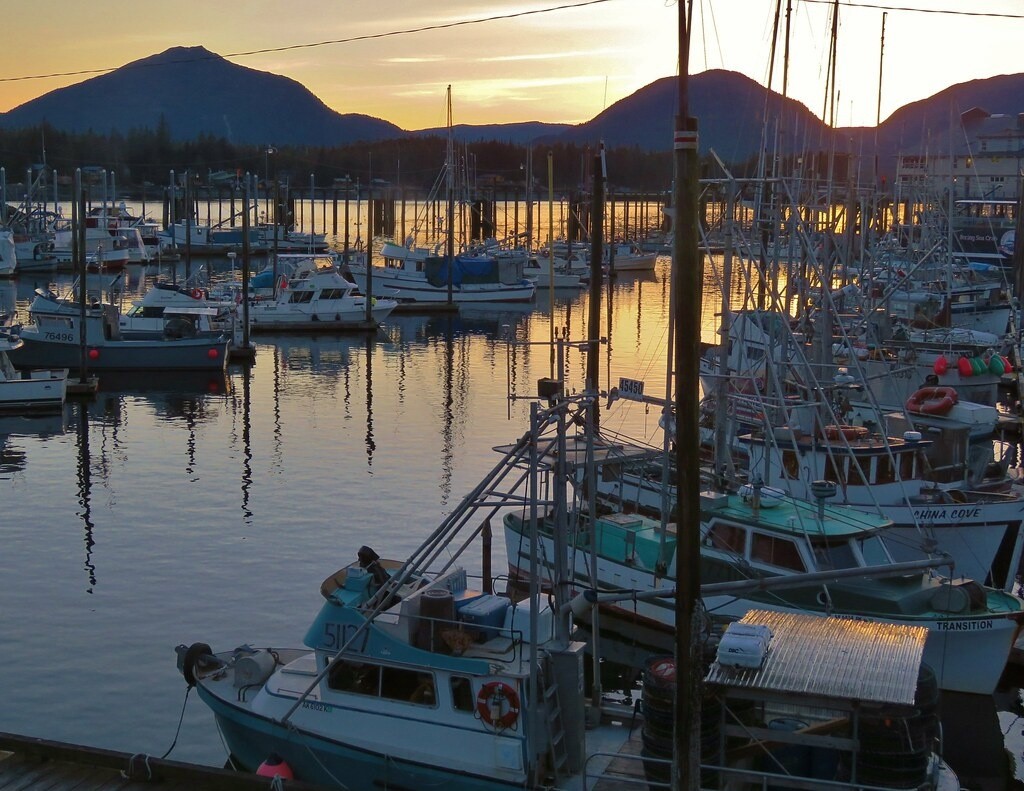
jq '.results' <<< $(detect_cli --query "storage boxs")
[700,491,729,509]
[774,426,803,441]
[452,588,488,626]
[457,595,511,644]
[718,622,774,669]
[738,483,787,508]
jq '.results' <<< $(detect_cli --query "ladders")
[527,648,572,787]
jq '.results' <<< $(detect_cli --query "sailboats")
[0,1,1024,791]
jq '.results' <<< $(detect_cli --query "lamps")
[536,377,565,409]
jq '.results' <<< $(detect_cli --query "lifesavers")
[192,288,203,299]
[477,681,520,728]
[906,386,957,412]
[820,424,867,441]
[543,250,549,258]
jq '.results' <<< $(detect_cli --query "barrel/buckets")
[766,663,936,788]
[640,651,723,791]
